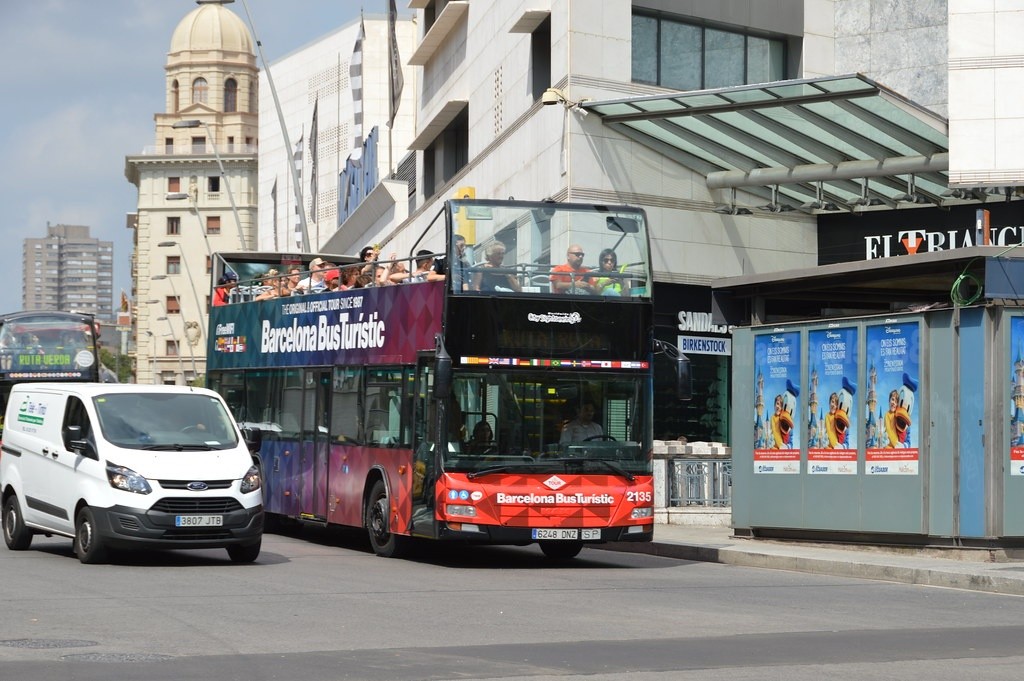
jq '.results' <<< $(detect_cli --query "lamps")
[713,186,1010,216]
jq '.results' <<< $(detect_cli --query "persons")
[213,246,434,307]
[427,235,522,293]
[1,331,47,370]
[769,378,800,449]
[820,376,857,449]
[882,372,919,448]
[469,421,493,447]
[885,390,900,448]
[549,245,629,296]
[825,392,839,449]
[771,394,783,449]
[559,398,602,445]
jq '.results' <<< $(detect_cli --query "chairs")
[228,274,552,304]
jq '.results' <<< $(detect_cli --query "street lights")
[196,0,311,252]
[158,241,206,343]
[152,274,198,379]
[146,299,188,385]
[172,120,248,250]
[166,192,212,258]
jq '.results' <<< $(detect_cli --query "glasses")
[351,273,361,276]
[365,252,371,258]
[456,243,467,248]
[569,252,584,258]
[602,258,616,263]
[480,427,490,432]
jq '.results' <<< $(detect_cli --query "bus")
[205,199,692,558]
[0,310,100,453]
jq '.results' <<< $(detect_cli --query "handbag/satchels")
[565,286,593,295]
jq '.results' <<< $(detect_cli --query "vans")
[0,381,264,566]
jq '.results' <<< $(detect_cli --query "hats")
[326,271,339,281]
[309,258,327,269]
[223,272,236,281]
